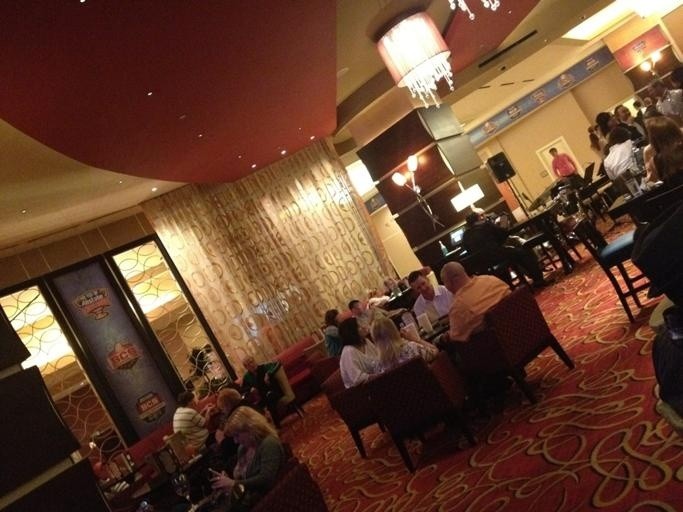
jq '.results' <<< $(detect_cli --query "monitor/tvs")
[449,226,465,246]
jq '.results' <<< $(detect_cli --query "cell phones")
[208,468,221,476]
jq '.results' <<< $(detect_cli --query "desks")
[609,176,681,224]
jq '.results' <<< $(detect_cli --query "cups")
[417,313,434,334]
[626,179,642,197]
[405,324,421,342]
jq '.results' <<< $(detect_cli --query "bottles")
[438,240,449,256]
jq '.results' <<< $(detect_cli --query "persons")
[588,61,683,181]
[172,390,215,451]
[240,354,285,430]
[440,261,514,350]
[201,388,288,512]
[549,147,580,184]
[462,207,554,288]
[407,270,455,328]
[322,275,440,389]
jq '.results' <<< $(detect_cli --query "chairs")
[254,218,652,512]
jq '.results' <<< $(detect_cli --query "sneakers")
[656,398,683,432]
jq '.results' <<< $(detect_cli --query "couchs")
[96,338,319,510]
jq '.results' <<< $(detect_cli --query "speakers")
[488,152,516,183]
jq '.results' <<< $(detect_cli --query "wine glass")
[171,475,197,512]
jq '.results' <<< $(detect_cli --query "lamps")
[374,5,455,109]
